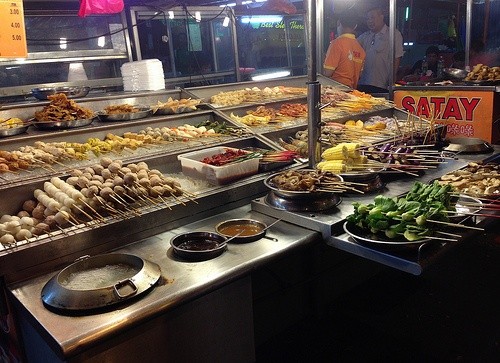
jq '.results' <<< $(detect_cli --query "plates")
[400,193,483,218]
[343,218,439,249]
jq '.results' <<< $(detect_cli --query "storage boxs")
[178,146,263,186]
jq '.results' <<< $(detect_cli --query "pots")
[41,254,163,314]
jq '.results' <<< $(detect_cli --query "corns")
[0,117,24,130]
[316,143,368,174]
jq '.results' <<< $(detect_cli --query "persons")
[408,37,486,82]
[322,15,366,90]
[357,8,404,98]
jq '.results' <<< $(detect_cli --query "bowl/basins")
[315,158,385,194]
[215,218,267,240]
[263,169,344,211]
[170,230,228,259]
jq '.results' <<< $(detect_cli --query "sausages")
[324,122,346,130]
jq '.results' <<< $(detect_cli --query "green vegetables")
[346,181,460,241]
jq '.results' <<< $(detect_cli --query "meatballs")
[1,157,184,245]
